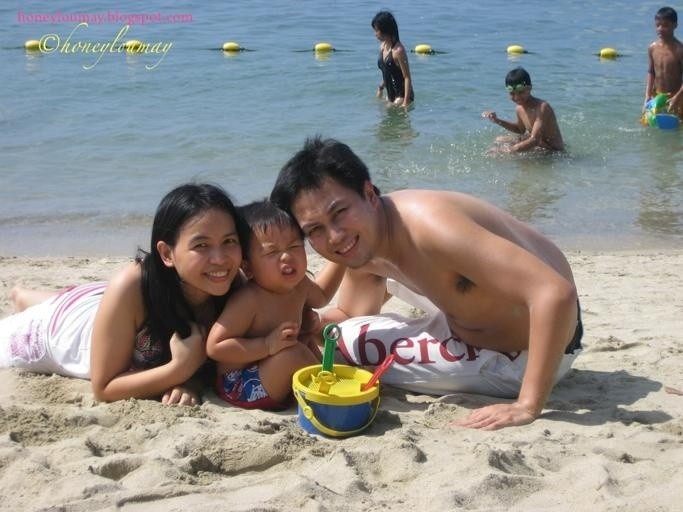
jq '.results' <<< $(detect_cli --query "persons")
[638,91,681,130]
[481,66,563,156]
[0,180,246,405]
[205,200,350,414]
[289,320,395,440]
[371,10,414,112]
[642,7,682,125]
[269,135,585,431]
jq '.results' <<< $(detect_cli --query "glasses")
[504,82,531,93]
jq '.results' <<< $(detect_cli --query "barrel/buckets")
[291,362,381,439]
